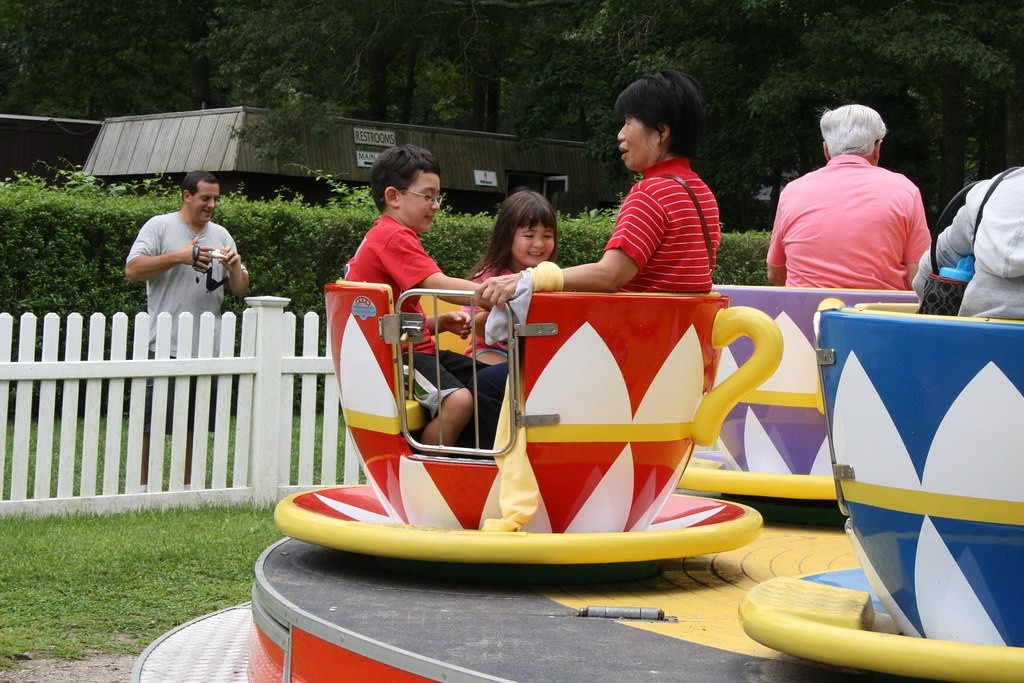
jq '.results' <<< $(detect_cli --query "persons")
[911,166,1024,323]
[466,68,722,459]
[344,144,494,458]
[461,189,558,367]
[766,104,932,290]
[124,170,250,494]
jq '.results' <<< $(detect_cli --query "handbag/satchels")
[915,167,1021,315]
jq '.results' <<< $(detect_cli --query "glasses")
[381,188,443,207]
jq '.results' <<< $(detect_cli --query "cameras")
[193,261,208,273]
